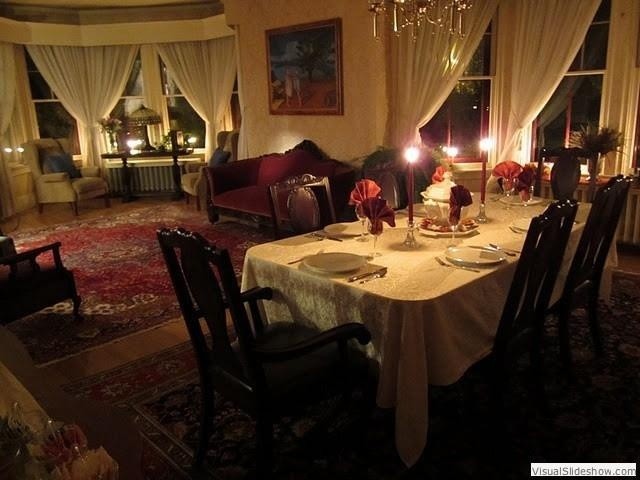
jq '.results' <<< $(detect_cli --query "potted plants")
[565,120,626,183]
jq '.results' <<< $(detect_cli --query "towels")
[344,155,540,236]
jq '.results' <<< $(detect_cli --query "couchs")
[202,138,356,226]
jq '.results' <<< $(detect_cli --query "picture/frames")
[263,14,346,117]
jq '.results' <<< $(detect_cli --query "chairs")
[0,231,83,329]
[541,172,635,366]
[410,197,580,480]
[21,136,112,216]
[532,143,602,203]
[152,220,385,478]
[268,171,338,241]
[179,130,240,212]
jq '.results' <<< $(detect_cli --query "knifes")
[310,232,343,243]
[346,266,387,281]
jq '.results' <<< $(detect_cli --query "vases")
[108,136,122,153]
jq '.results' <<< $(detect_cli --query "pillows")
[46,153,82,179]
[207,145,232,170]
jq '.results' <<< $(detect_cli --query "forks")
[360,269,387,284]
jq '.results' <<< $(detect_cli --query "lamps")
[127,101,164,152]
[366,0,476,45]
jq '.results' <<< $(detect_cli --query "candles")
[406,140,416,224]
[480,137,488,201]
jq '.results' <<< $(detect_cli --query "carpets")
[45,263,639,480]
[1,200,303,372]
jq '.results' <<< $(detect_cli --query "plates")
[400,170,577,273]
[322,221,367,238]
[302,252,366,275]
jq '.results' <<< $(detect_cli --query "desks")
[0,361,117,480]
[98,145,195,206]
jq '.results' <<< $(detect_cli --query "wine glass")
[364,217,386,260]
[355,203,372,244]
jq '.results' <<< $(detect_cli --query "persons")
[305,41,321,82]
[281,40,304,108]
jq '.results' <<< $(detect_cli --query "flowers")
[97,115,124,137]
